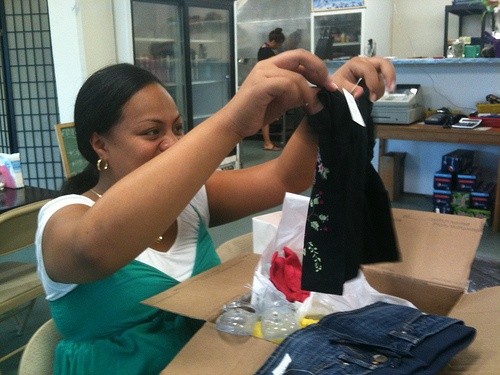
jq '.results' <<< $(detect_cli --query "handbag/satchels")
[249,190,417,322]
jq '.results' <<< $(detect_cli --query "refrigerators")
[310,0,366,88]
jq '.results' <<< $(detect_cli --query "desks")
[372,114,500,232]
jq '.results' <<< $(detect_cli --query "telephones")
[425,107,450,123]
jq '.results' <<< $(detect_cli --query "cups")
[465,45,481,58]
[448,45,464,58]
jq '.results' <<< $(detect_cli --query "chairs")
[0,200,64,375]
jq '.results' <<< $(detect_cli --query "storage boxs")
[380,151,407,203]
[139,192,488,375]
[463,44,480,58]
[476,102,500,116]
[432,149,496,226]
[470,116,500,127]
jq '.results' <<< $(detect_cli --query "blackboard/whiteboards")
[55,123,90,179]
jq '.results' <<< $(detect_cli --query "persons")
[35,48,395,375]
[257,28,285,150]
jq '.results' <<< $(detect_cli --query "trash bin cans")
[379,152,407,202]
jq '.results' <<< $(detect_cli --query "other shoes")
[264,146,281,151]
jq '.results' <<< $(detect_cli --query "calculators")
[451,117,482,129]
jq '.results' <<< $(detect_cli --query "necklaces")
[90,189,163,244]
